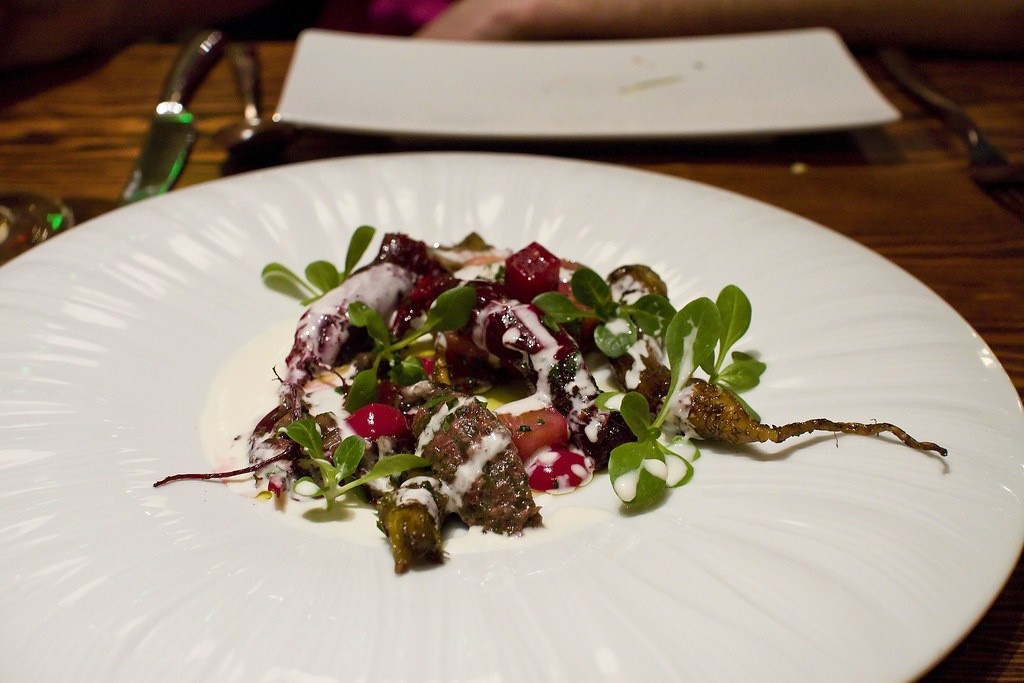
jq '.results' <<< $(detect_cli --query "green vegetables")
[261,224,765,513]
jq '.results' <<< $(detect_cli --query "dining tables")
[0,44,1024,683]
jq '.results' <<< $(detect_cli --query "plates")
[0,150,1024,683]
[275,29,901,143]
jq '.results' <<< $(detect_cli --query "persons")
[0,0,1024,88]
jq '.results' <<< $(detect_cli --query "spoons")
[214,48,288,148]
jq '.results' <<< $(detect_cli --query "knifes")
[116,30,221,212]
